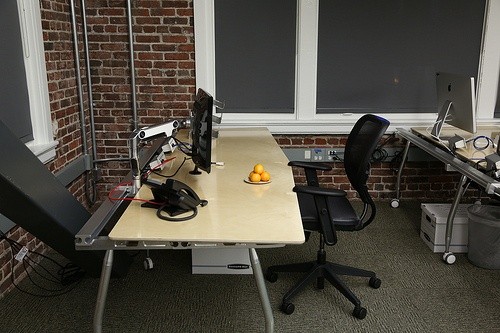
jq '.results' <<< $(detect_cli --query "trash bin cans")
[466,203,500,270]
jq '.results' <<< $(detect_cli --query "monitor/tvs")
[430,72,477,148]
[192,88,213,174]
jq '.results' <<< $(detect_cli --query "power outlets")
[327,151,338,161]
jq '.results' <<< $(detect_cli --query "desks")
[390,128,500,264]
[74,127,306,333]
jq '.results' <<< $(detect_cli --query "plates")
[245,177,272,183]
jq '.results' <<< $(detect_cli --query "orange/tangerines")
[249,163,269,182]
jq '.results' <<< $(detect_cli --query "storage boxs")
[192,249,254,274]
[420,203,475,253]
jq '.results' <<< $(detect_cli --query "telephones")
[150,178,208,216]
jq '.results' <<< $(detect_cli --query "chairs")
[265,113,390,319]
